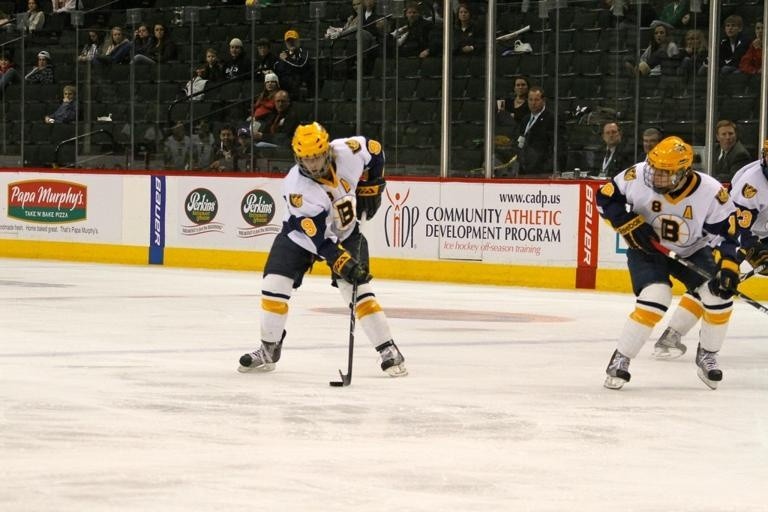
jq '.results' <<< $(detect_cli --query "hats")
[229,38,243,47]
[284,30,300,41]
[37,51,50,58]
[265,73,280,89]
[238,128,250,137]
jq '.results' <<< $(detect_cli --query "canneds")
[574,168,580,180]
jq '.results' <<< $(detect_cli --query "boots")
[240,329,286,367]
[376,340,404,370]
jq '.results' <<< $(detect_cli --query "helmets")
[292,121,330,178]
[643,136,693,194]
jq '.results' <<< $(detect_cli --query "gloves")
[356,178,386,220]
[744,242,768,276]
[708,255,739,299]
[327,249,373,285]
[612,211,660,255]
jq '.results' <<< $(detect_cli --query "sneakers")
[696,343,722,380]
[655,326,686,354]
[606,351,630,382]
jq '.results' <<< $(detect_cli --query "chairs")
[0,2,763,178]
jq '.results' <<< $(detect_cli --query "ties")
[719,151,725,163]
[605,149,610,164]
[529,117,534,126]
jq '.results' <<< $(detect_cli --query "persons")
[239,118,405,371]
[654,136,768,355]
[597,137,741,382]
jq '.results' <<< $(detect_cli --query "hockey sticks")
[339,210,367,385]
